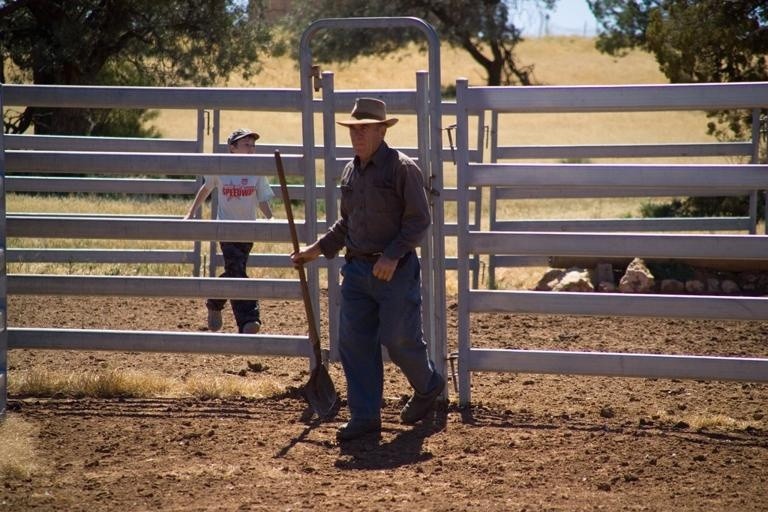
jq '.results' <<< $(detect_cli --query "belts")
[345,250,412,264]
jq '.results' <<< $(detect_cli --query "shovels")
[274,149,339,418]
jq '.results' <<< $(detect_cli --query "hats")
[336,97,399,127]
[227,128,260,146]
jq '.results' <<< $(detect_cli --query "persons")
[175,129,277,334]
[287,93,445,442]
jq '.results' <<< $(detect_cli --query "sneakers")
[241,320,261,334]
[400,373,445,422]
[207,309,222,332]
[336,416,381,442]
